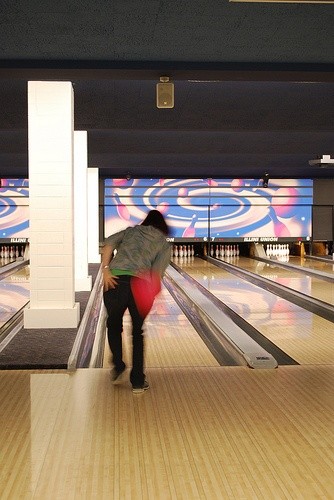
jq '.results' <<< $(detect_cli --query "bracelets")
[102,265,110,269]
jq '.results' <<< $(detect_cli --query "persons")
[102,210,172,393]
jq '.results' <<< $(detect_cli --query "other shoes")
[111,361,126,380]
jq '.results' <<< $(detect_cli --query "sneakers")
[133,381,149,393]
